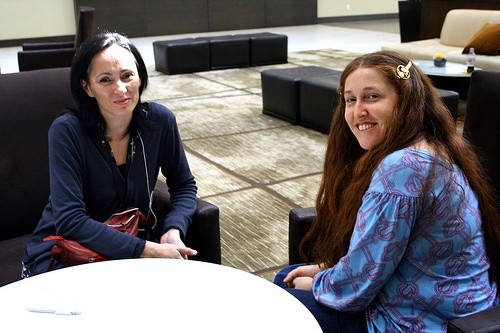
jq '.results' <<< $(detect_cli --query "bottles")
[467,48,476,73]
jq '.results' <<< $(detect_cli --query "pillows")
[462,23,500,56]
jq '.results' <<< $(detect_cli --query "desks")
[0,258,323,333]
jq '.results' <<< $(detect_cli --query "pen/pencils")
[24,308,82,316]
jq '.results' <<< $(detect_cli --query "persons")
[21,32,198,275]
[272,50,500,333]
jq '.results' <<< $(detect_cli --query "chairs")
[289,70,500,333]
[18,6,95,71]
[0,67,221,287]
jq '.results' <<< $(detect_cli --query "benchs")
[382,9,500,71]
[153,32,288,74]
[261,66,459,135]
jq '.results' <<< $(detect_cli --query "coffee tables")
[412,60,482,96]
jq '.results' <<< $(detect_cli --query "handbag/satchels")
[51,207,148,268]
